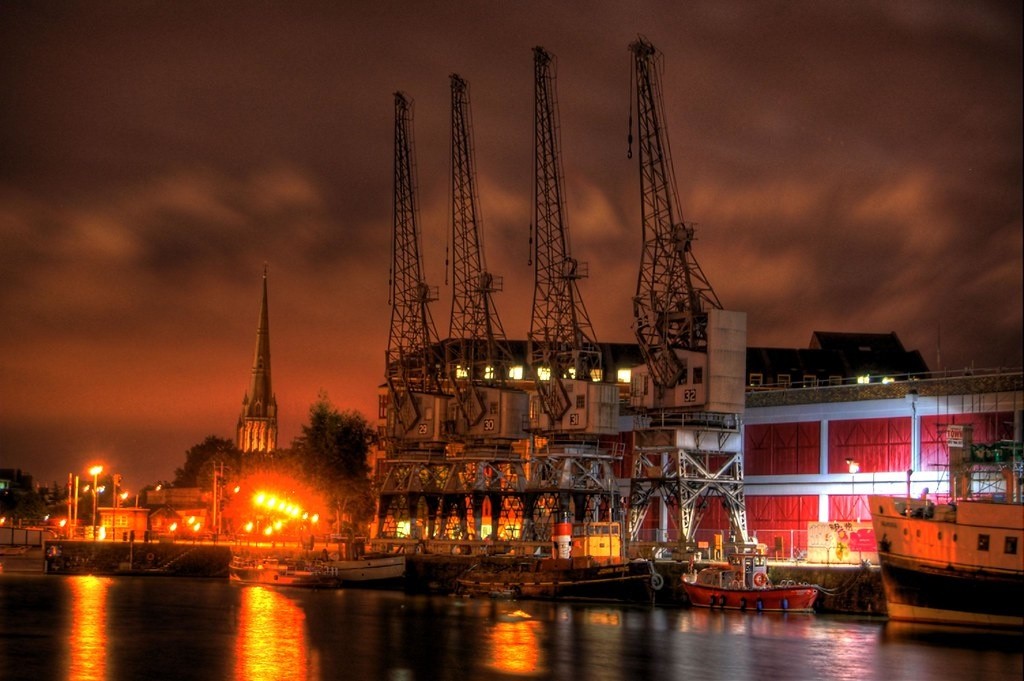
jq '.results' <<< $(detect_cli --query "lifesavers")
[753,571,768,587]
[650,574,665,591]
[146,552,155,560]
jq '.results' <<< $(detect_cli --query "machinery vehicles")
[358,35,753,563]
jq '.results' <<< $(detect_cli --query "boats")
[455,507,664,606]
[308,548,407,584]
[227,553,325,587]
[866,419,1024,646]
[677,538,819,613]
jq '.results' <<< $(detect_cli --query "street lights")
[88,465,102,541]
